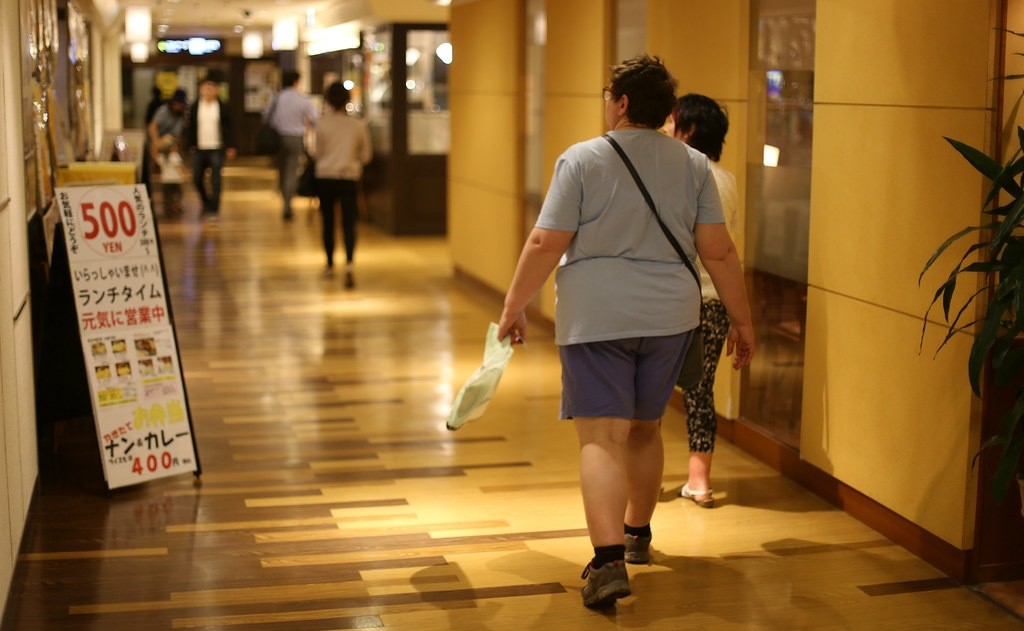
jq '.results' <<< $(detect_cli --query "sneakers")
[622,532,653,564]
[580,557,631,605]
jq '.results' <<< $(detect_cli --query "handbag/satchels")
[269,132,280,154]
[446,321,515,429]
[298,161,318,197]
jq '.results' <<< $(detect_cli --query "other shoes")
[347,271,355,288]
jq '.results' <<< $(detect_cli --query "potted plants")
[916,122,1024,517]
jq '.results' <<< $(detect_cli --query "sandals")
[677,482,714,508]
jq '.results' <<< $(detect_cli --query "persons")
[150,89,189,217]
[663,94,738,508]
[497,55,756,608]
[263,71,316,220]
[147,87,163,122]
[180,78,235,219]
[311,82,372,289]
